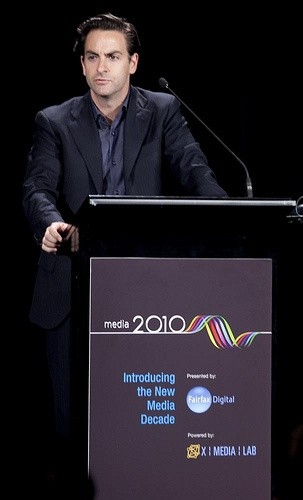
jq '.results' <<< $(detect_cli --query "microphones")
[159,78,253,198]
